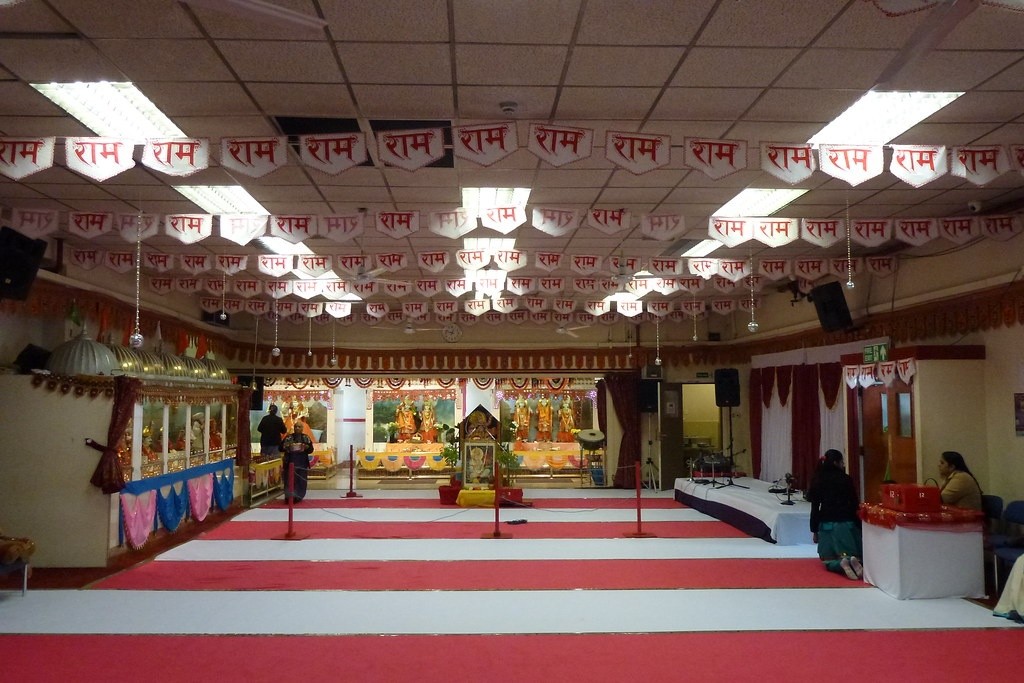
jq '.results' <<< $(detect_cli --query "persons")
[192,414,205,453]
[419,401,438,442]
[513,399,530,442]
[176,430,186,451]
[209,417,222,448]
[257,405,287,458]
[937,451,983,512]
[556,398,575,442]
[142,428,158,461]
[283,400,309,443]
[469,449,489,478]
[278,422,313,501]
[157,428,174,453]
[536,397,554,442]
[396,397,417,442]
[806,449,863,580]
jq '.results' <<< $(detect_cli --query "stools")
[0,536,37,598]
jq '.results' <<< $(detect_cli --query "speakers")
[237,376,264,410]
[715,367,740,406]
[202,310,229,328]
[638,380,659,413]
[810,281,853,333]
[0,226,48,304]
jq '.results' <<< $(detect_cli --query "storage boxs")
[438,484,461,505]
[881,483,940,512]
[499,487,523,503]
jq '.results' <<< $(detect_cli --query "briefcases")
[881,483,942,512]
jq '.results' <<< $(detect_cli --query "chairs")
[981,494,1024,599]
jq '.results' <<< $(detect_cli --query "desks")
[858,501,987,600]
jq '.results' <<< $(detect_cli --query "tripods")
[717,406,750,489]
[640,412,659,493]
[703,452,725,487]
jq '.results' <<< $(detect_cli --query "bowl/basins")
[290,443,301,451]
